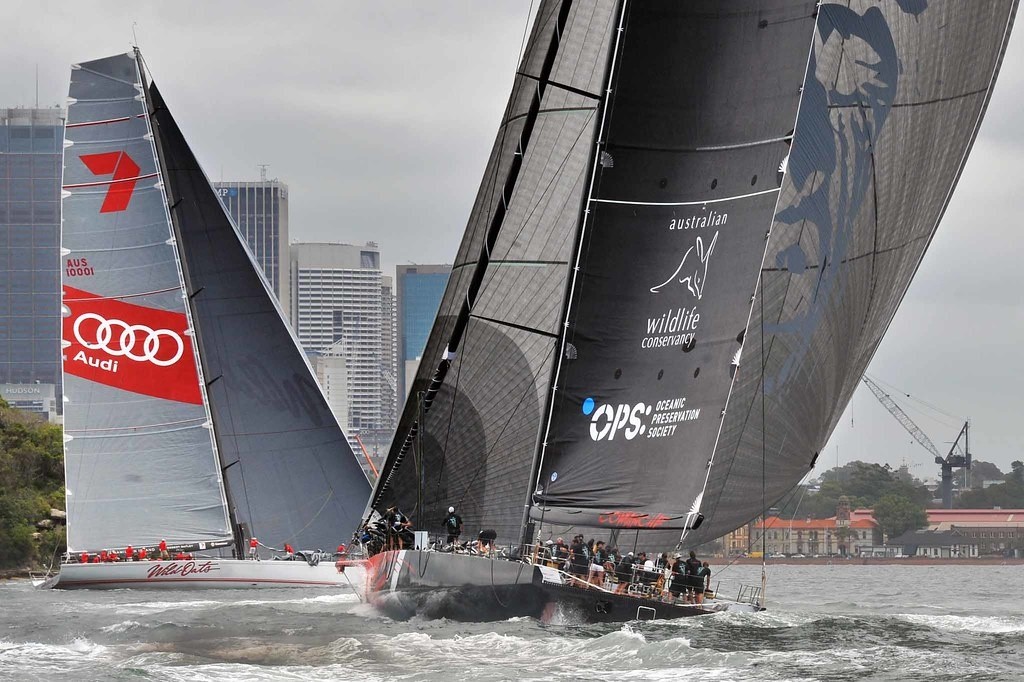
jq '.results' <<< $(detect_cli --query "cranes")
[859,371,973,508]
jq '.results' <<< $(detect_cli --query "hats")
[341,543,345,546]
[162,538,166,541]
[627,552,633,556]
[448,507,455,513]
[672,553,683,559]
[142,547,145,550]
[128,544,132,547]
[251,537,257,540]
[545,539,553,545]
[596,541,606,544]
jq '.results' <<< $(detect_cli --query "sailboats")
[25,39,385,591]
[333,0,1021,628]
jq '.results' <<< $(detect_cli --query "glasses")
[601,544,604,545]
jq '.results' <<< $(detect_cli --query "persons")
[284,543,294,561]
[379,507,412,552]
[249,538,257,553]
[442,507,463,550]
[534,534,710,610]
[159,539,170,559]
[336,543,347,556]
[175,551,193,560]
[125,545,150,562]
[81,550,117,563]
[477,530,497,554]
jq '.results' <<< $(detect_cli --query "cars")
[790,553,805,558]
[769,553,787,559]
